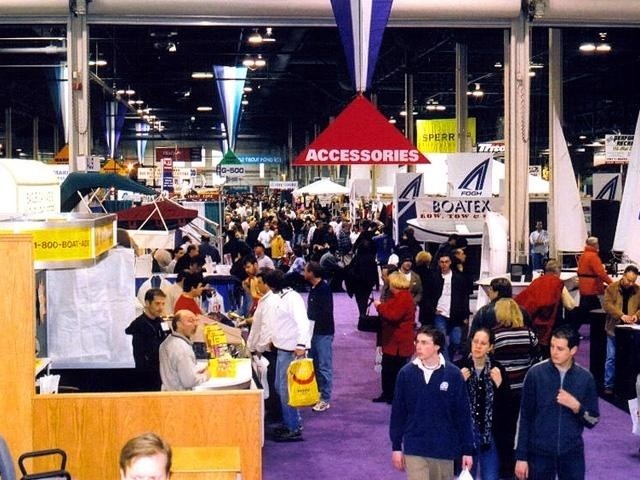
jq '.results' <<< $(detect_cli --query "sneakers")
[272,427,303,442]
[313,399,330,413]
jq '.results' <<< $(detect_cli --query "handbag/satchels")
[287,354,320,408]
[356,301,381,333]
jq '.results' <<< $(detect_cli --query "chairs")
[35,357,52,376]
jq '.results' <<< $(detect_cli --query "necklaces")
[420,358,441,370]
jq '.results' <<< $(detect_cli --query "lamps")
[87,49,166,143]
[246,27,276,47]
[386,31,612,124]
[242,53,266,71]
[243,80,253,93]
[69,0,93,18]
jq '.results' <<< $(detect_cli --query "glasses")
[414,340,434,346]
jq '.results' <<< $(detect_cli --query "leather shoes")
[372,394,392,405]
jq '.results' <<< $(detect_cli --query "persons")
[517,261,575,361]
[453,327,511,480]
[120,434,172,480]
[604,265,640,397]
[463,278,530,361]
[530,220,550,269]
[513,327,600,479]
[176,193,395,316]
[577,237,614,338]
[371,226,470,404]
[390,325,475,480]
[126,235,334,442]
[493,298,543,441]
